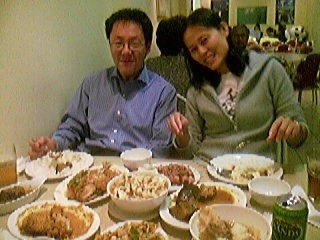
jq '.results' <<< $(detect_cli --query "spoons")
[192,149,233,178]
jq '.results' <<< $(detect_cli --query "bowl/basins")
[120,148,153,170]
[107,170,171,213]
[188,204,273,240]
[248,176,292,207]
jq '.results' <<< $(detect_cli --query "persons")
[165,7,312,165]
[28,8,177,160]
[250,13,291,44]
[230,24,251,56]
[145,14,194,117]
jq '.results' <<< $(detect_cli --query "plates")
[207,153,284,187]
[7,199,101,240]
[138,162,200,194]
[158,181,247,229]
[53,164,130,204]
[108,200,160,222]
[25,150,94,181]
[100,218,169,240]
[0,177,47,216]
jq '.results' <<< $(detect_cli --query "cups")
[307,150,320,210]
[0,144,18,188]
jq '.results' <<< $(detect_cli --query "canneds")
[271,193,309,240]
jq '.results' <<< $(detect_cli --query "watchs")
[292,120,302,133]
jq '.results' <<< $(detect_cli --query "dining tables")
[259,52,308,86]
[1,156,320,240]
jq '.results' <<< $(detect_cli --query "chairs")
[145,56,198,159]
[291,53,320,105]
